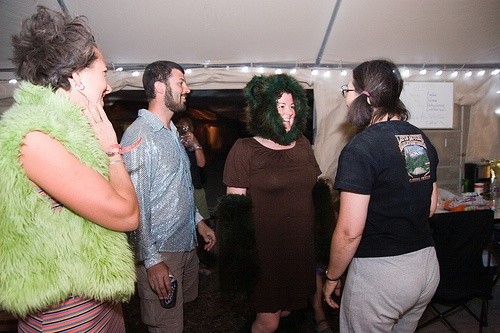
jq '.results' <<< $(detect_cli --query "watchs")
[323,268,340,281]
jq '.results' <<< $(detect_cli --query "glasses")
[341,84,360,97]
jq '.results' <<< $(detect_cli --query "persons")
[171,110,213,278]
[323,59,440,333]
[216,73,337,333]
[119,60,216,333]
[0,5,140,333]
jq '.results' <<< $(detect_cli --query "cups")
[474,183,488,198]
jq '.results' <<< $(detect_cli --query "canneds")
[160,275,177,309]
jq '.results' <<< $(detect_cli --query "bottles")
[490,177,500,212]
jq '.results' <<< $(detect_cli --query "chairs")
[415,209,495,333]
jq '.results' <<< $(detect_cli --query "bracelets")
[103,136,143,165]
[193,146,202,150]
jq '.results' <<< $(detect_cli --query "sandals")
[314,318,331,331]
